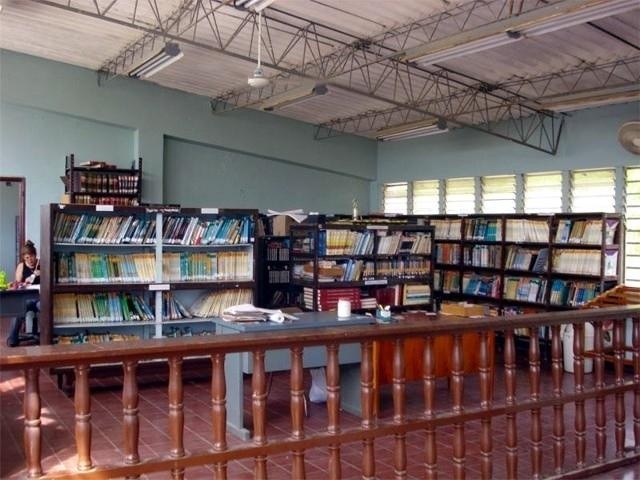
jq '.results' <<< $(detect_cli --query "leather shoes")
[7,340,18,346]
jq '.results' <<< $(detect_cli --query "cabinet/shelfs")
[258,212,434,312]
[433,212,624,367]
[50,203,258,390]
[62,153,143,205]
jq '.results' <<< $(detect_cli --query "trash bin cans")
[26,311,39,332]
[563,321,594,373]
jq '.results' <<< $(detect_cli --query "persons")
[7,237,43,347]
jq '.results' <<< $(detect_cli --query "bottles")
[336,297,353,318]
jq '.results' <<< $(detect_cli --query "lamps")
[257,80,327,116]
[397,0,640,76]
[373,118,454,149]
[128,43,185,79]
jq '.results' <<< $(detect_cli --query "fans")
[615,119,639,155]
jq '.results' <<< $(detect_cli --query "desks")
[210,300,495,440]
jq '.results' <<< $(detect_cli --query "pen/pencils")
[377,303,391,310]
